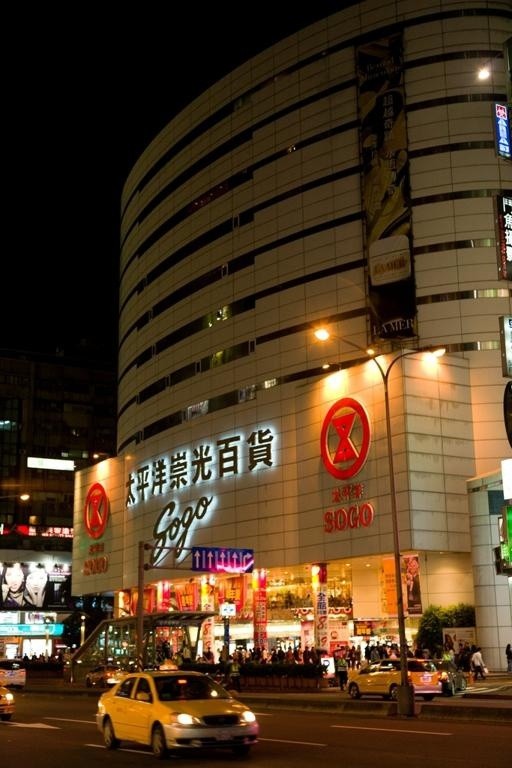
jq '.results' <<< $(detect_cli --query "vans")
[0,658,28,692]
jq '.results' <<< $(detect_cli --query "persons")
[23,563,50,608]
[233,644,319,667]
[180,639,191,660]
[1,562,29,612]
[405,556,421,606]
[203,647,214,664]
[216,644,229,661]
[230,658,242,690]
[504,643,512,672]
[161,639,173,658]
[466,647,487,681]
[61,648,73,684]
[336,654,349,690]
[339,636,482,672]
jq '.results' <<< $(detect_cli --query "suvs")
[344,656,445,702]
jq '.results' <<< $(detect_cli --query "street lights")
[219,598,237,689]
[308,324,450,721]
[44,617,51,664]
[79,615,87,647]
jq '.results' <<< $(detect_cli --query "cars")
[85,664,130,689]
[94,658,262,762]
[0,686,17,721]
[427,658,469,699]
